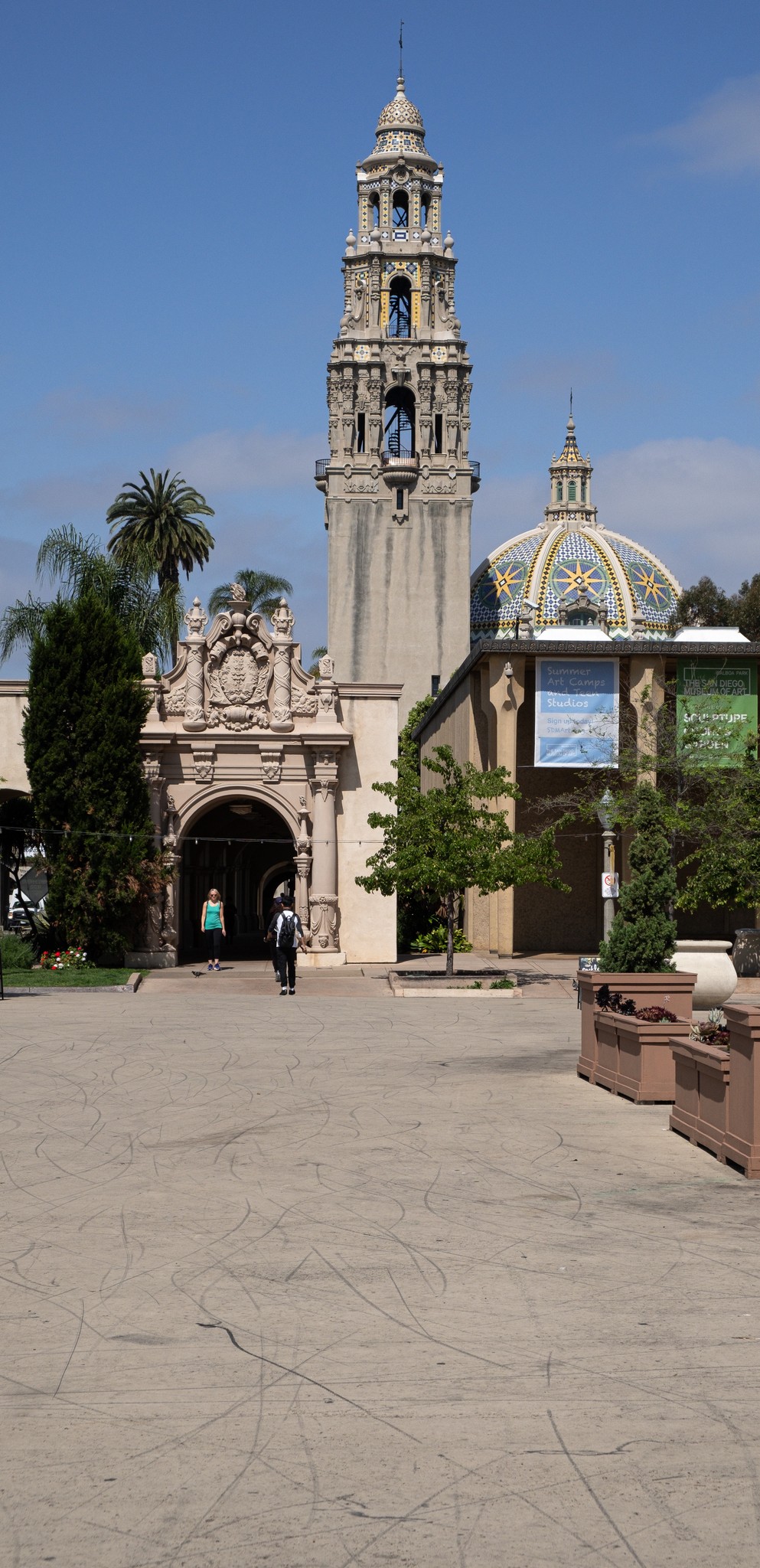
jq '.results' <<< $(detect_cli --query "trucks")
[7,889,46,934]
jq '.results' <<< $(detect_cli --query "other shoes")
[279,989,288,996]
[288,989,295,995]
[275,970,280,982]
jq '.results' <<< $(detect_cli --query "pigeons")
[191,971,207,978]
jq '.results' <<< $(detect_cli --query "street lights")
[596,787,619,947]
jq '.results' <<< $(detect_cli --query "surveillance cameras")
[505,668,513,679]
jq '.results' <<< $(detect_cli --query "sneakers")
[214,963,221,970]
[207,963,213,970]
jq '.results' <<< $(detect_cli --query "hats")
[274,897,282,905]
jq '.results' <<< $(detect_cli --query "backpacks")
[278,912,297,947]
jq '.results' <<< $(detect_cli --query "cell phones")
[223,932,225,937]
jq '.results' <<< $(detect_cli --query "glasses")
[210,894,217,896]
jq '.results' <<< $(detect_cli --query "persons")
[263,893,307,995]
[201,889,226,970]
